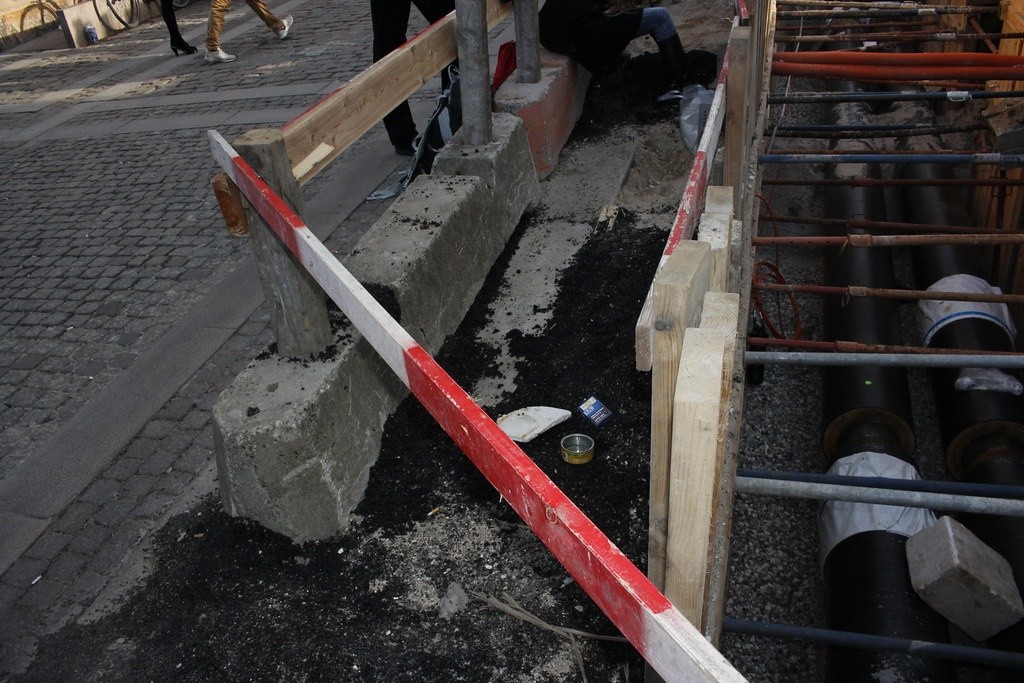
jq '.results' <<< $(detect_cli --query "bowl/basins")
[561,434,594,464]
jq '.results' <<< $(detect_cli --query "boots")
[657,34,686,77]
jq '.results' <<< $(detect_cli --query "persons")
[159,0,197,57]
[370,2,517,198]
[203,0,294,64]
[537,0,720,99]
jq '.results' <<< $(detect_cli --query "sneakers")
[204,46,236,62]
[277,15,295,39]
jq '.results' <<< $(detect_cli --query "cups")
[88,27,98,44]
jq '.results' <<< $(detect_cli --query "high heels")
[171,39,198,56]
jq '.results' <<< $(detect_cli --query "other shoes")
[396,132,423,154]
